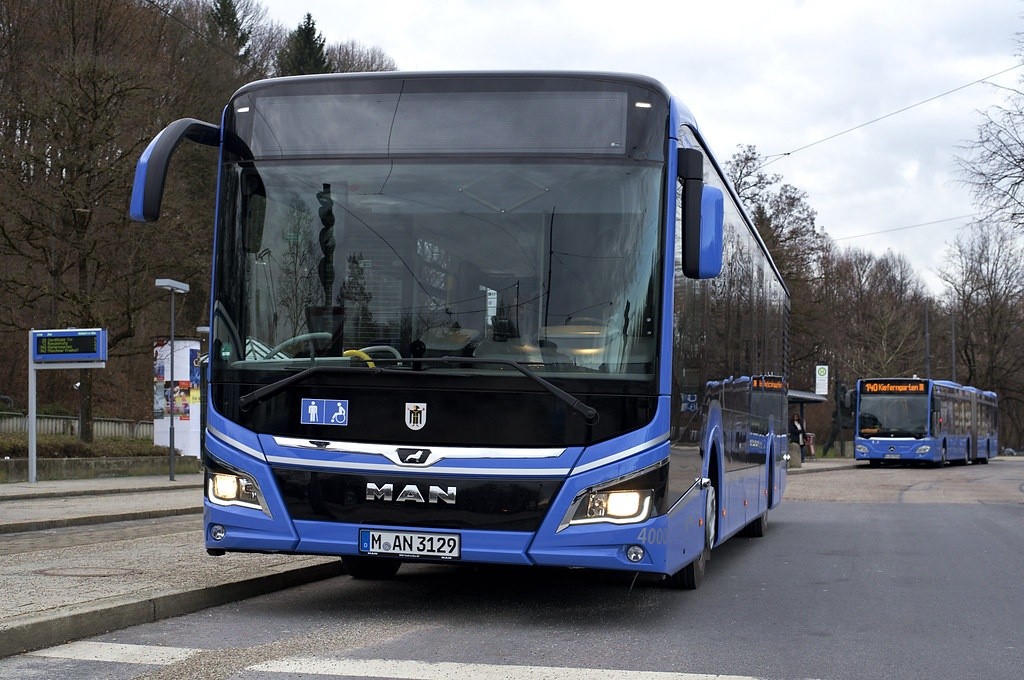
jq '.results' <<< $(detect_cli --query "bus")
[125,64,797,599]
[845,374,999,470]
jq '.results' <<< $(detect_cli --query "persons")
[557,279,637,335]
[788,413,808,463]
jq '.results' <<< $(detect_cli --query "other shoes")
[801,460,805,463]
